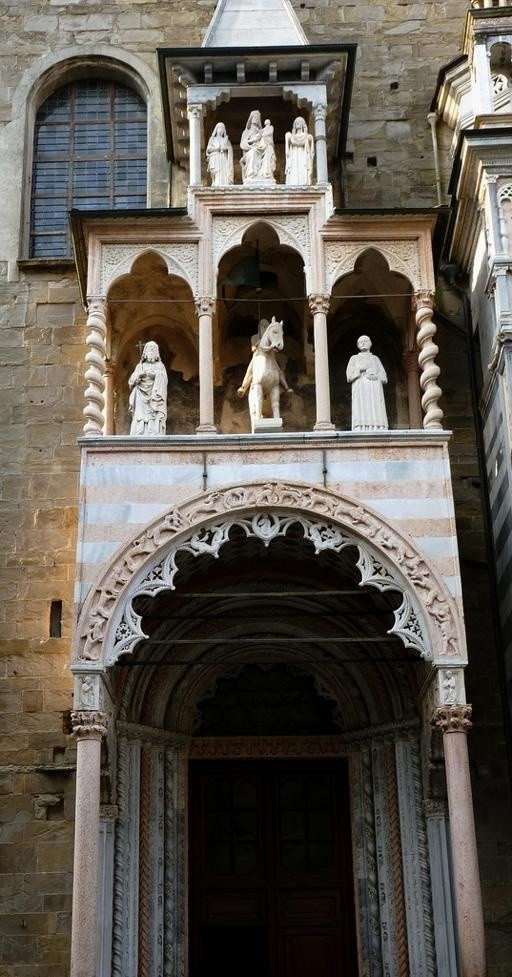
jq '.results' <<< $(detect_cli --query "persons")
[259,118,274,148]
[128,338,169,437]
[204,118,236,189]
[284,116,315,188]
[239,111,277,187]
[236,314,296,398]
[345,335,391,434]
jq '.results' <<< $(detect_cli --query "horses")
[248,316,284,434]
